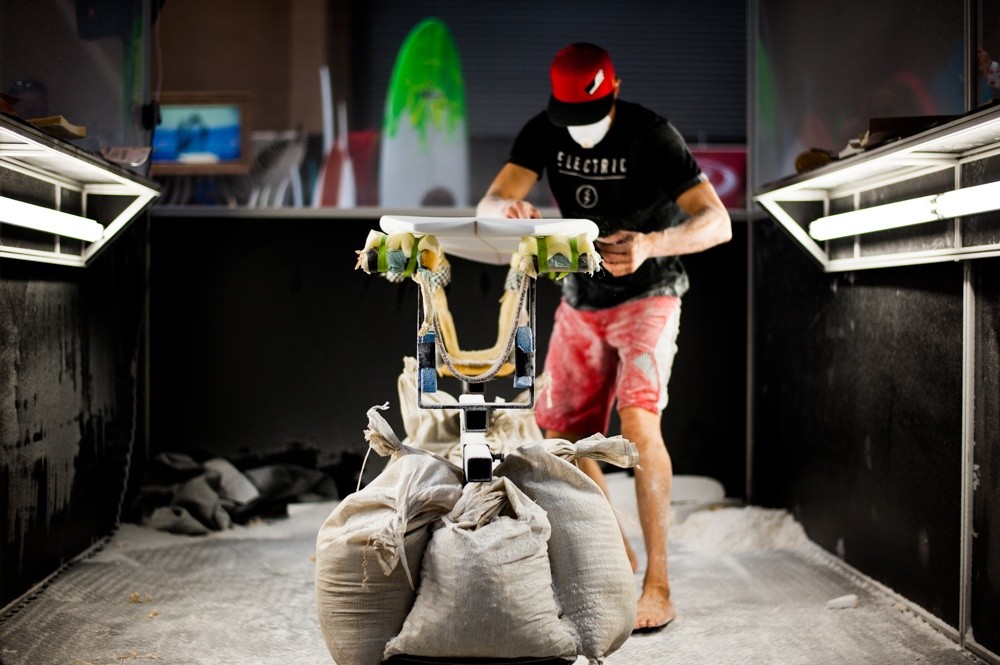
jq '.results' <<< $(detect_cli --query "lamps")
[0,195,106,242]
[808,180,1000,241]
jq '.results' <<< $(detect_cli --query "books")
[26,115,86,141]
[102,146,151,167]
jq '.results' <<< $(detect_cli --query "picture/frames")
[153,90,255,175]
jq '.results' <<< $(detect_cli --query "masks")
[568,115,611,150]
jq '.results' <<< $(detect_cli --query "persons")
[476,40,732,629]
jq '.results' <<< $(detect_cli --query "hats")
[546,42,617,125]
